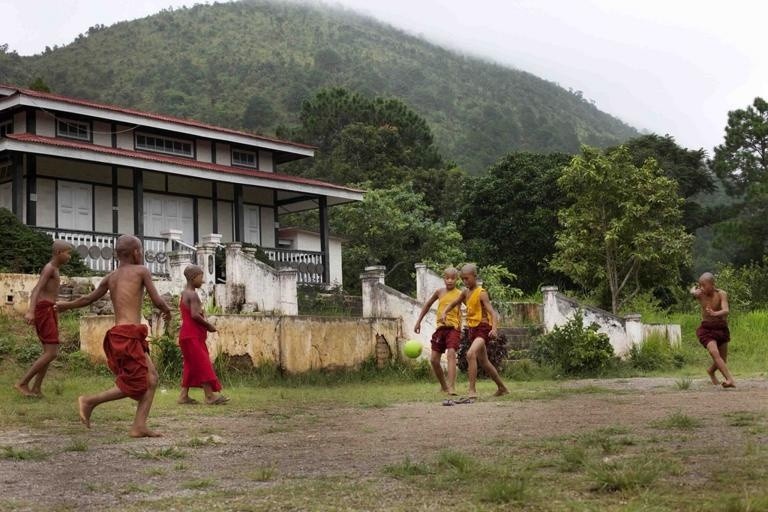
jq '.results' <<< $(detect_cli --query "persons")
[440,264,510,398]
[14,239,72,398]
[53,234,172,438]
[177,266,230,404]
[690,272,736,388]
[414,268,466,395]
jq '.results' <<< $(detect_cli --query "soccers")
[405,340,423,359]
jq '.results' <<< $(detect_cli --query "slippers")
[442,397,474,406]
